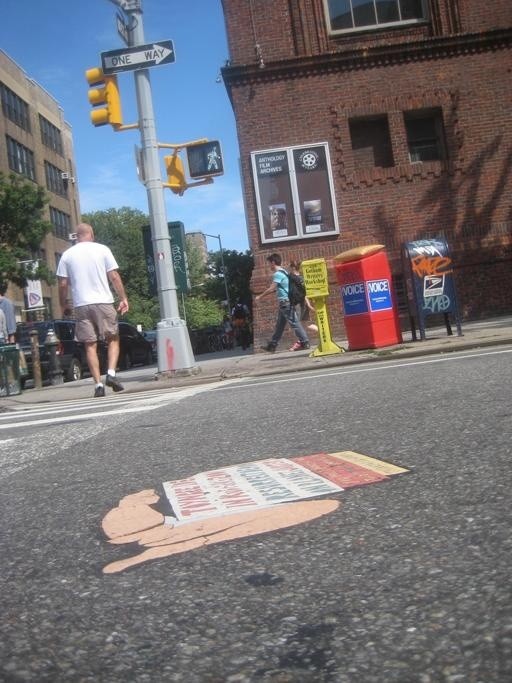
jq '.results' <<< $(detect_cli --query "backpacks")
[275,269,307,304]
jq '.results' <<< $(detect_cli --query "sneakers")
[94,385,106,396]
[105,374,124,392]
[260,343,277,353]
[288,340,309,352]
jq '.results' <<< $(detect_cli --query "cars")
[95,322,158,371]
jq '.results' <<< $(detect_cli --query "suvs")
[14,319,105,388]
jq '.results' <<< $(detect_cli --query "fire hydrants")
[44,329,65,386]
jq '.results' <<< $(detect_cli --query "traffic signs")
[100,38,176,77]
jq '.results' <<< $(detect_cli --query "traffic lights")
[84,65,123,128]
[185,139,224,180]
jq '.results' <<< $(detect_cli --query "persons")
[254,252,310,352]
[56,221,130,397]
[0,278,24,397]
[0,307,14,396]
[286,258,317,353]
[232,295,253,349]
[220,314,233,346]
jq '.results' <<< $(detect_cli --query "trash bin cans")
[0,343,22,396]
